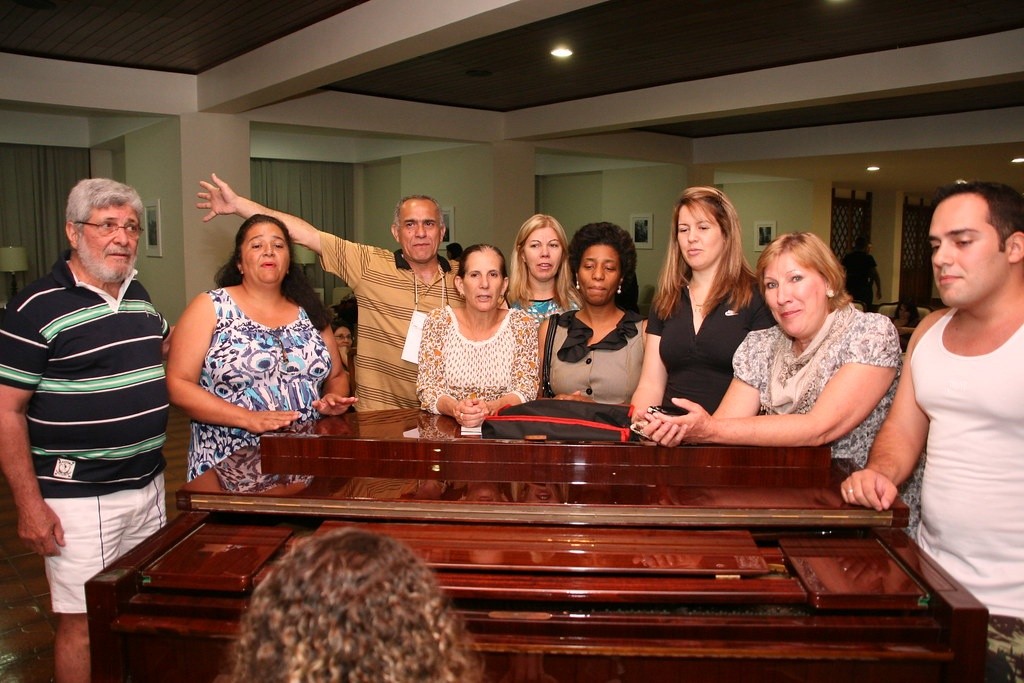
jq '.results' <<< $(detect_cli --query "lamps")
[0,246,29,308]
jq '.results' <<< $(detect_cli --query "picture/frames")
[144,199,162,257]
[631,213,654,250]
[437,205,455,250]
[753,220,778,252]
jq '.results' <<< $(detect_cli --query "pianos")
[82,408,990,683]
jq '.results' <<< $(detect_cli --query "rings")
[847,489,853,493]
[459,413,464,420]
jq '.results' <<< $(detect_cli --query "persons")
[887,298,921,353]
[195,172,466,413]
[628,185,778,444]
[848,240,882,313]
[416,243,540,429]
[185,418,318,496]
[165,213,360,483]
[1,178,178,683]
[506,214,583,322]
[643,229,929,541]
[331,321,355,354]
[446,242,465,260]
[839,183,1023,683]
[214,528,486,683]
[331,409,924,593]
[535,221,649,404]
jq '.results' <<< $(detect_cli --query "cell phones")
[648,405,688,417]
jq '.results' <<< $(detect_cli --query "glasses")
[334,334,355,341]
[73,219,146,238]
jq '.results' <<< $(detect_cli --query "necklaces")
[689,287,703,313]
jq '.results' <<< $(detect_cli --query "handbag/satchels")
[481,397,635,445]
